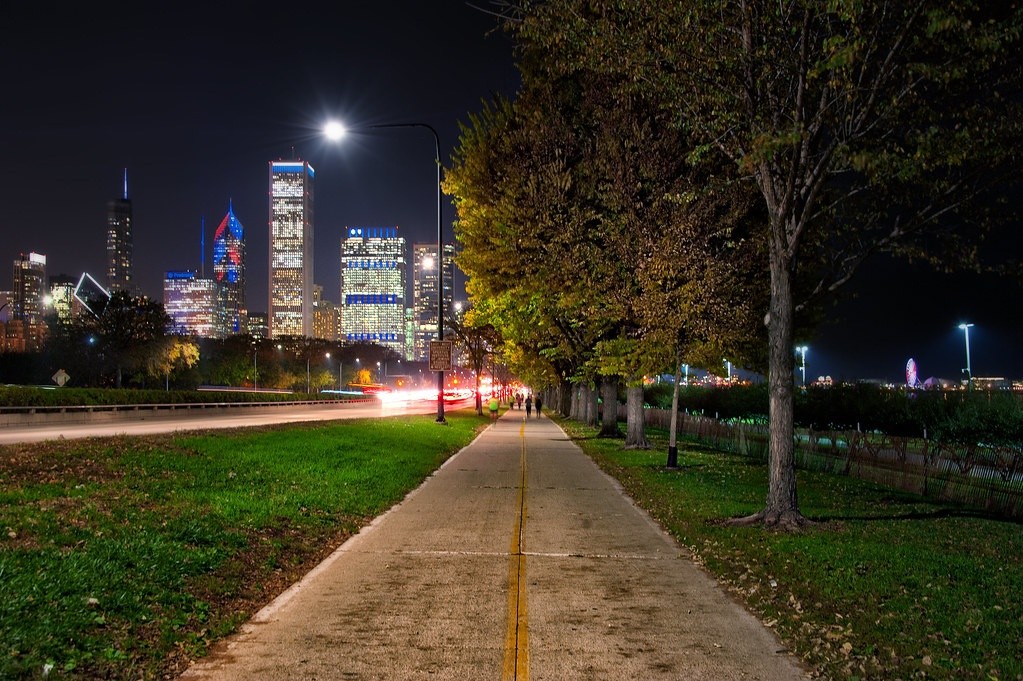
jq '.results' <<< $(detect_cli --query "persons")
[534,392,543,419]
[516,393,531,409]
[486,395,501,426]
[508,393,515,410]
[524,396,531,418]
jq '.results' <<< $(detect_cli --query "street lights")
[321,117,449,425]
